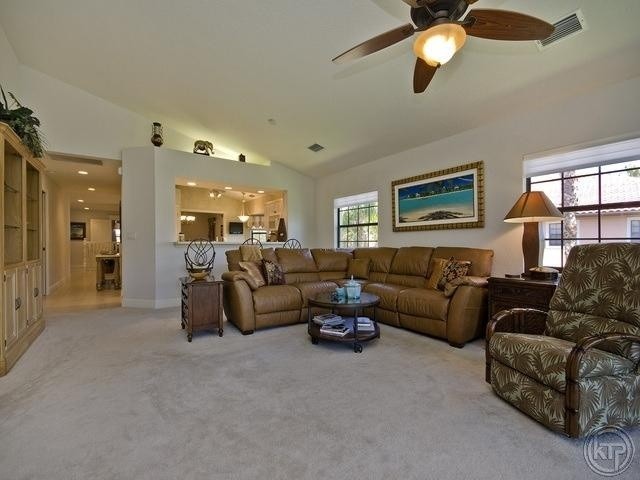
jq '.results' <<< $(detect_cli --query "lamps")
[413,23,466,69]
[209,189,224,199]
[503,192,566,276]
[238,193,250,222]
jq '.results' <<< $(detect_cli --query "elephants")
[193,140,215,155]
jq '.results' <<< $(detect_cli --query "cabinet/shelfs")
[0,121,47,377]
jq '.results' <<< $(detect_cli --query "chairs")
[485,242,639,437]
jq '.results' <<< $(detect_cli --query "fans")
[333,0,555,94]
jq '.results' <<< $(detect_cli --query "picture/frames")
[70,222,86,240]
[392,161,485,232]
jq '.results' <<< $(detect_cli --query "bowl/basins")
[188,270,211,280]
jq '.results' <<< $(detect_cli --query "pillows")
[261,260,285,284]
[239,260,265,288]
[347,258,371,279]
[428,256,447,289]
[437,256,467,291]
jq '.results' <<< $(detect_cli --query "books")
[312,313,375,337]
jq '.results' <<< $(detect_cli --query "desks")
[487,275,561,335]
[96,252,120,290]
[181,276,224,342]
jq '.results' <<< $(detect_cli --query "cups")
[335,283,361,300]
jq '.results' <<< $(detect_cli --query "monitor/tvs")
[230,222,243,234]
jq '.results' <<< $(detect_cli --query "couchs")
[223,246,493,349]
